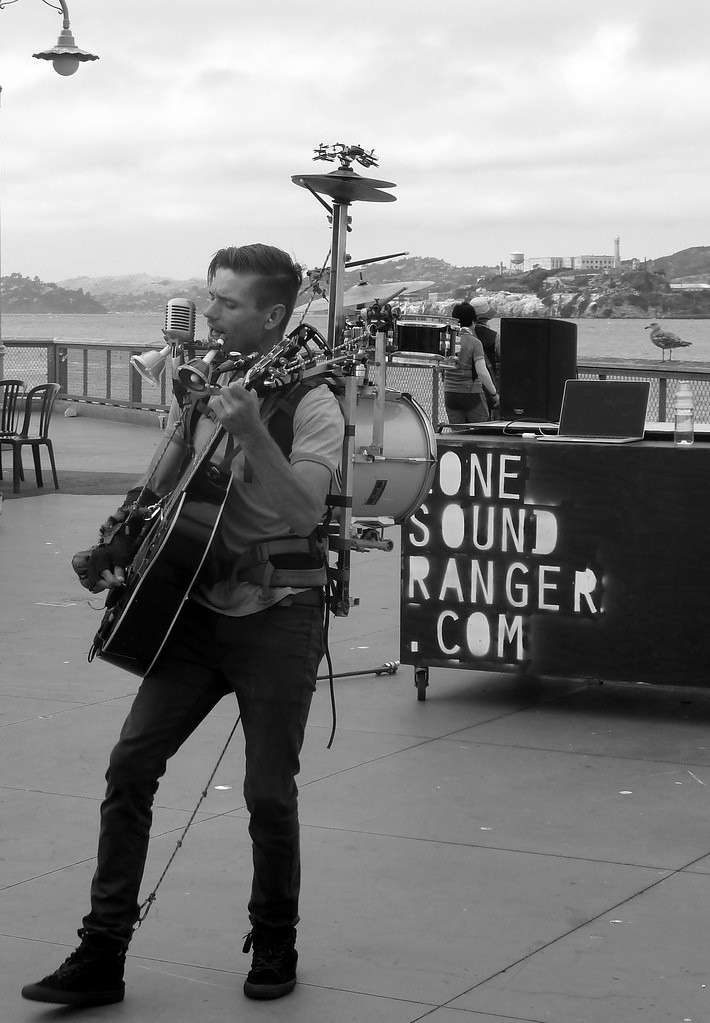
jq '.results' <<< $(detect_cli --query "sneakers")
[21,927,128,1007]
[242,915,298,1000]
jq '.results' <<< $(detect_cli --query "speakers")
[500,318,577,423]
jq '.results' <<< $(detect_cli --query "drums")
[369,316,462,370]
[329,385,439,519]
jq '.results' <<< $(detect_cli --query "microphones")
[162,298,196,409]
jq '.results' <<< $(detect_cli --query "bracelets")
[491,393,497,396]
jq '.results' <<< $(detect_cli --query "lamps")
[131,345,172,384]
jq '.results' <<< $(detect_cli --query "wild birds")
[643,322,693,362]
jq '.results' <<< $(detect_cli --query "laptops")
[537,380,652,443]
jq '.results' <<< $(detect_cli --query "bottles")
[674,381,694,446]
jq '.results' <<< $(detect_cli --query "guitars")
[87,322,316,674]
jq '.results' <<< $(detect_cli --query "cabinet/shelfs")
[400,434,709,701]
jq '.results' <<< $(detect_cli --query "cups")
[158,416,165,429]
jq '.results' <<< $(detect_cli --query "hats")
[469,297,497,319]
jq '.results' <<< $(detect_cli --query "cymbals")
[292,170,396,205]
[295,281,436,312]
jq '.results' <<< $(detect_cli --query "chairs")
[0,383,62,494]
[0,379,27,481]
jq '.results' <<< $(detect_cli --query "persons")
[21,243,346,1005]
[471,297,501,419]
[431,302,500,431]
